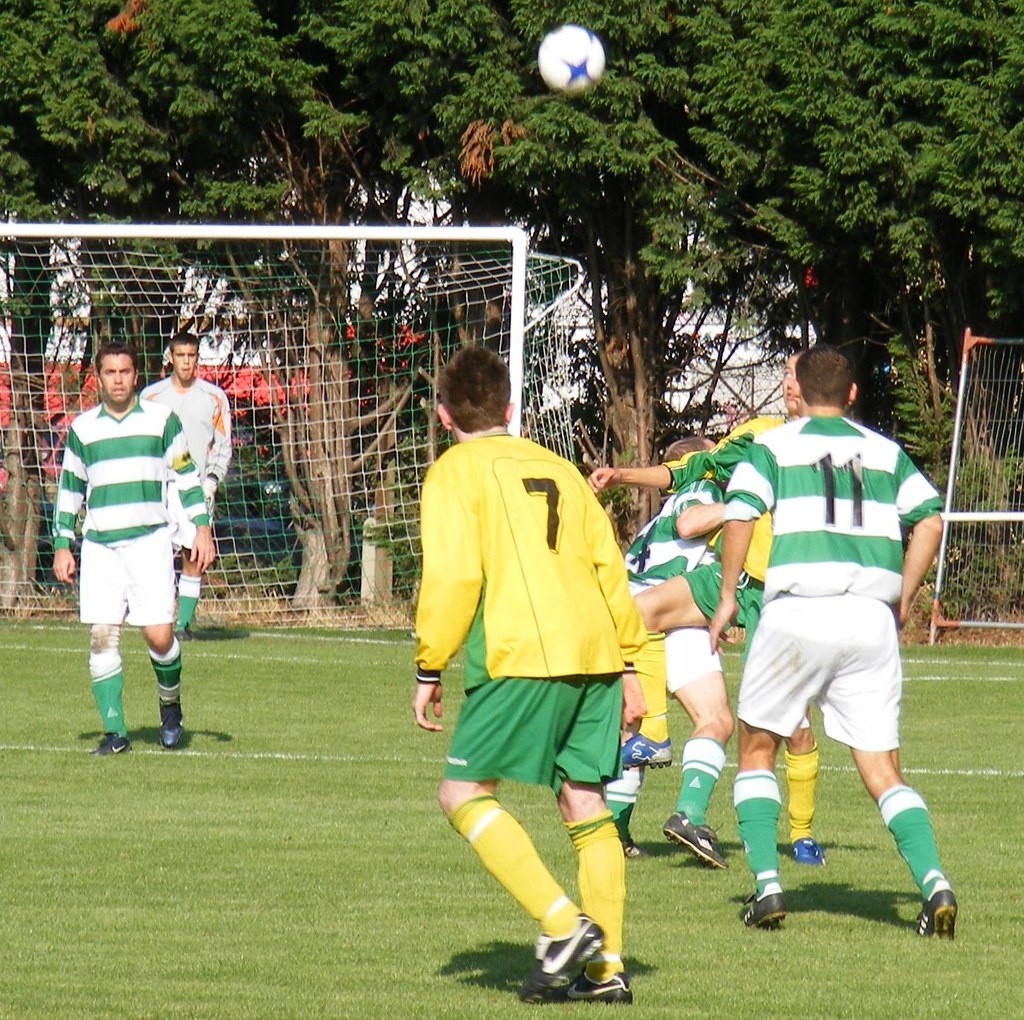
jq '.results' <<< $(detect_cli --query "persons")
[711,345,957,941]
[411,344,648,1004]
[140,333,233,640]
[50,342,215,755]
[601,436,736,868]
[589,350,826,863]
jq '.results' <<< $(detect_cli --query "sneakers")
[521,913,606,1004]
[88,732,132,758]
[620,835,647,860]
[742,892,786,929]
[917,890,957,942]
[159,697,182,749]
[792,839,825,866]
[620,734,674,771]
[544,966,634,1005]
[663,812,728,870]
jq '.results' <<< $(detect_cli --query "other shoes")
[174,630,193,642]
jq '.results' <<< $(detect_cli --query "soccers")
[536,21,609,97]
